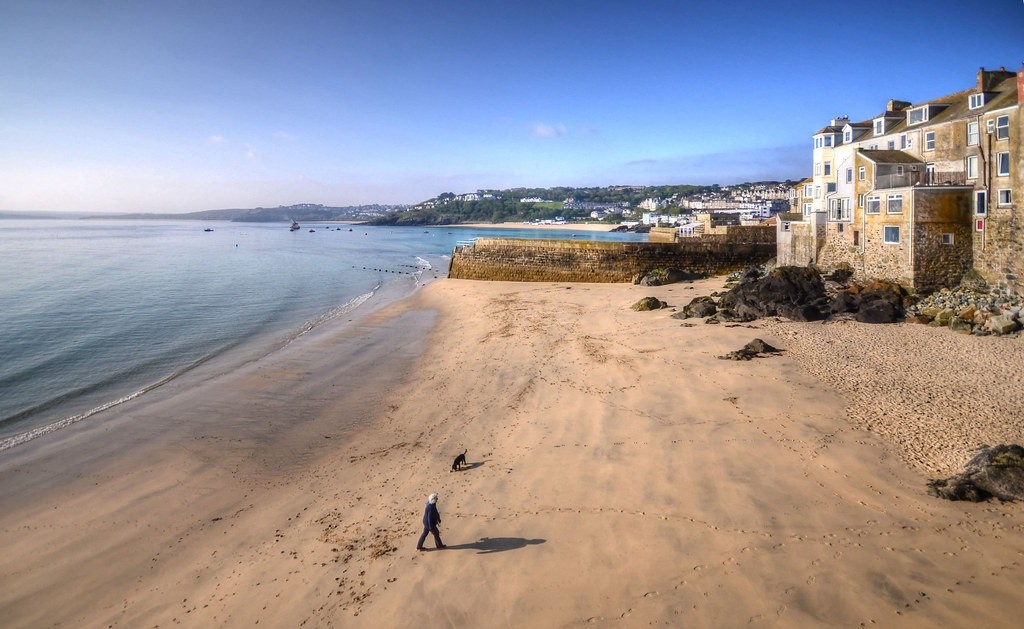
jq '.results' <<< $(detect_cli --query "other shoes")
[437,545,447,549]
[416,547,426,550]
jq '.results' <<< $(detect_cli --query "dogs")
[452,449,467,472]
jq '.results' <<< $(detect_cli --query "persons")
[417,494,446,550]
